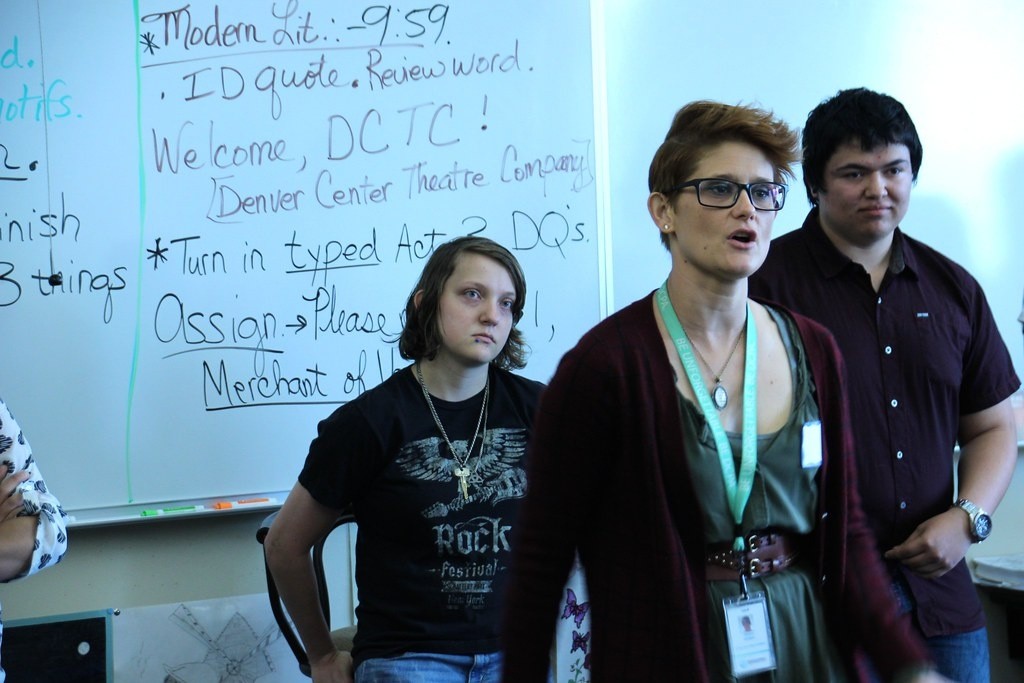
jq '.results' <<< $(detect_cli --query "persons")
[742,616,751,631]
[0,398,69,683]
[748,88,1021,683]
[264,236,546,683]
[502,101,953,683]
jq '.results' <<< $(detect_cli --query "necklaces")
[416,359,490,474]
[683,327,746,410]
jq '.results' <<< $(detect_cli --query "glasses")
[677,178,789,211]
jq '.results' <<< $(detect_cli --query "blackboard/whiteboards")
[0,0,613,525]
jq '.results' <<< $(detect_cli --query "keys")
[455,468,470,500]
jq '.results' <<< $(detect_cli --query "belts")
[701,533,796,581]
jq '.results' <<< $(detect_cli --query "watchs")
[951,498,993,543]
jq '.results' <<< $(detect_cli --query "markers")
[139,505,204,519]
[214,497,270,511]
